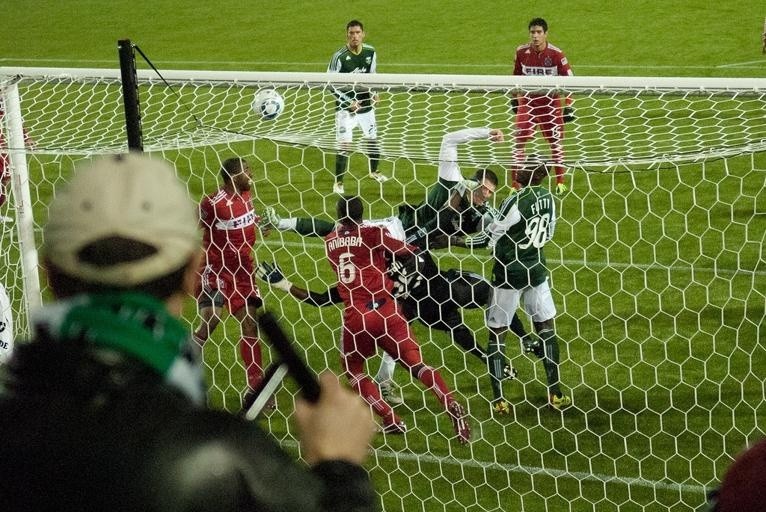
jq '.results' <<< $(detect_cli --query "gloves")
[564,106,576,122]
[254,261,294,293]
[436,234,457,247]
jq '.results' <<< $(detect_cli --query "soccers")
[254,90,286,120]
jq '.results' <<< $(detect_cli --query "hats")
[47,152,201,286]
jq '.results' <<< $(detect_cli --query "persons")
[434,152,572,414]
[261,127,502,406]
[325,20,388,195]
[0,151,383,512]
[323,195,471,444]
[509,16,575,196]
[183,157,278,411]
[255,247,541,380]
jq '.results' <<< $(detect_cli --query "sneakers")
[556,183,565,196]
[365,339,571,446]
[259,206,278,231]
[369,171,387,182]
[333,182,345,194]
